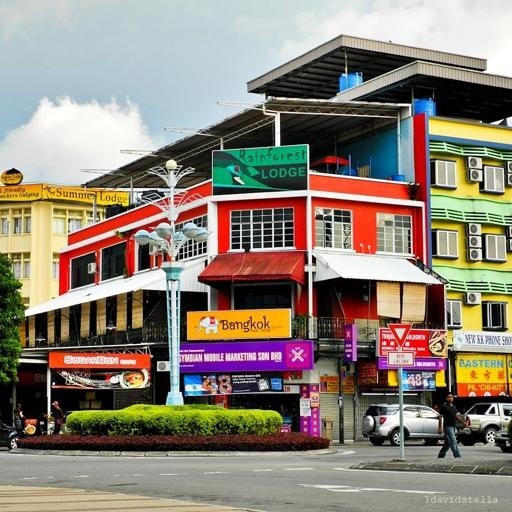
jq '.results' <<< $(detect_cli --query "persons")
[37,332,44,339]
[14,404,24,437]
[108,320,114,327]
[52,401,65,434]
[438,392,468,459]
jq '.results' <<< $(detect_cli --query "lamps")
[35,313,80,346]
[106,298,148,331]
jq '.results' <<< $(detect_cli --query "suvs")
[459,402,512,445]
[361,402,458,447]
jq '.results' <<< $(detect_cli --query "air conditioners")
[466,223,482,262]
[466,156,483,182]
[88,263,96,274]
[506,226,512,251]
[464,293,481,304]
[505,161,512,185]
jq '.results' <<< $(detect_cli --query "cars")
[0,419,23,451]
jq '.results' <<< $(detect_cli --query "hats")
[51,401,58,406]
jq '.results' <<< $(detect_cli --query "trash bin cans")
[321,417,333,441]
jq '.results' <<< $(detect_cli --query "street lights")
[134,157,213,407]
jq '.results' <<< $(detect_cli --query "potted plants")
[293,312,311,338]
